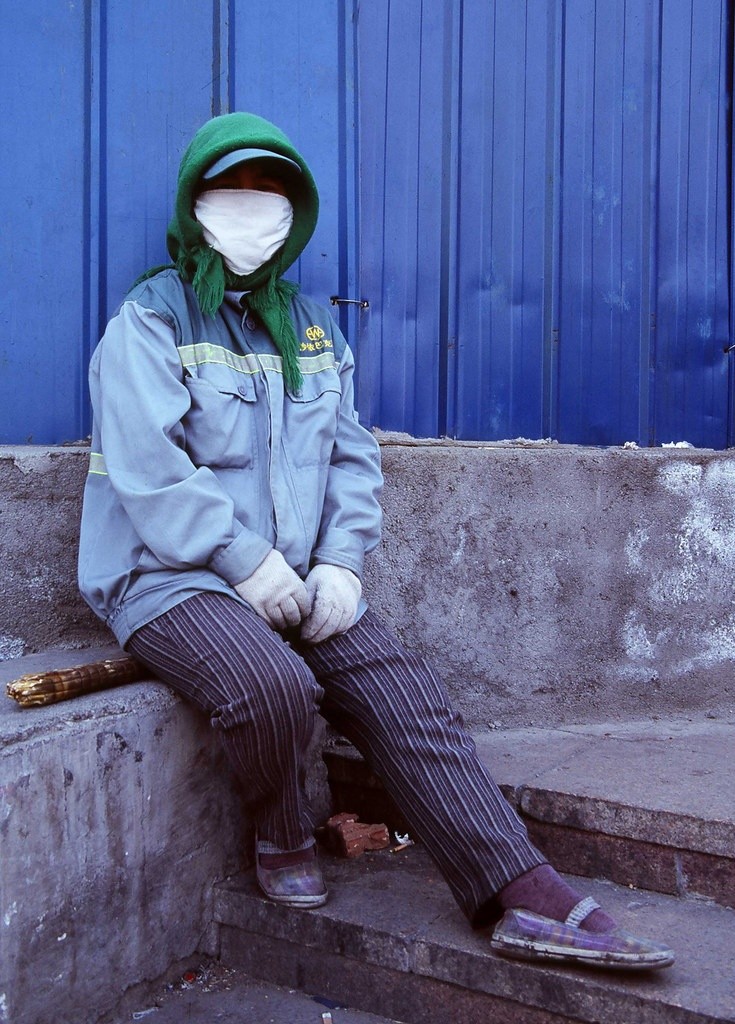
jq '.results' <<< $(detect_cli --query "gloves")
[233,548,311,630]
[296,563,362,642]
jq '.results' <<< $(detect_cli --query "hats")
[200,148,302,181]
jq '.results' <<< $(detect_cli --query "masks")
[190,189,294,277]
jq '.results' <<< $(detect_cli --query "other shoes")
[253,829,329,908]
[490,895,676,970]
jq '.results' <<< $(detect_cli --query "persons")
[77,111,678,972]
